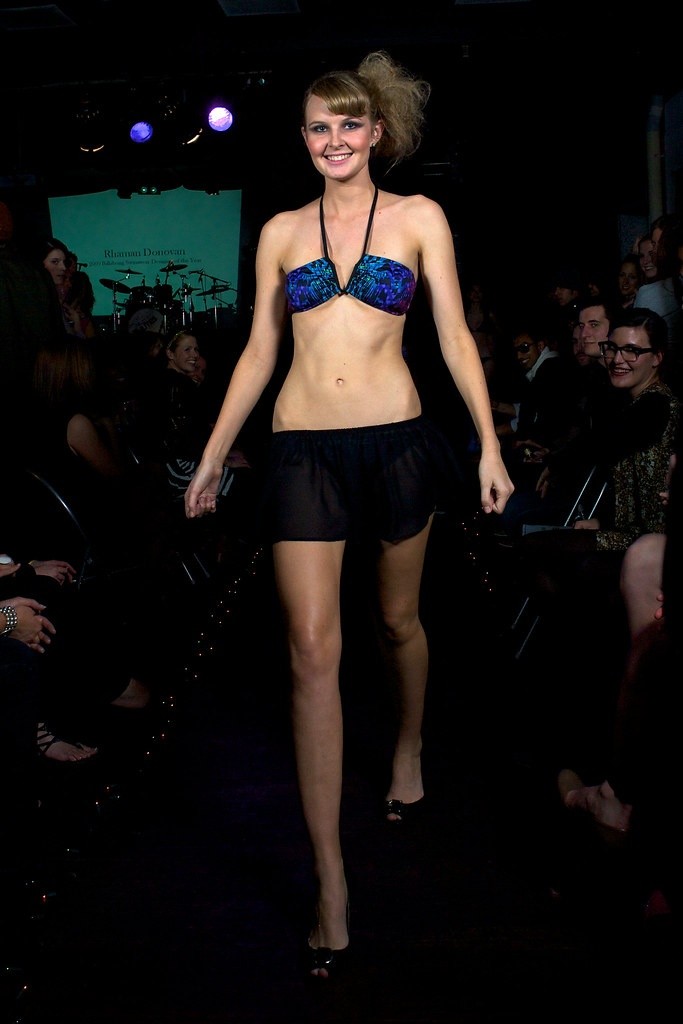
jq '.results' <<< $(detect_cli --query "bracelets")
[0,606,18,639]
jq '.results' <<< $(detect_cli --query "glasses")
[480,355,493,364]
[597,340,654,362]
[513,340,536,354]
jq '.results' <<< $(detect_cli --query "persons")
[184,50,514,986]
[476,216,683,910]
[0,238,249,768]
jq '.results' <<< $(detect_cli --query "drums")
[127,284,183,320]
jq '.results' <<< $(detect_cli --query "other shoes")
[35,723,98,764]
[101,683,177,718]
[303,897,354,978]
[382,793,427,831]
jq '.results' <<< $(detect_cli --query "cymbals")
[99,279,131,294]
[115,270,143,275]
[207,288,227,294]
[196,292,211,297]
[159,264,187,272]
